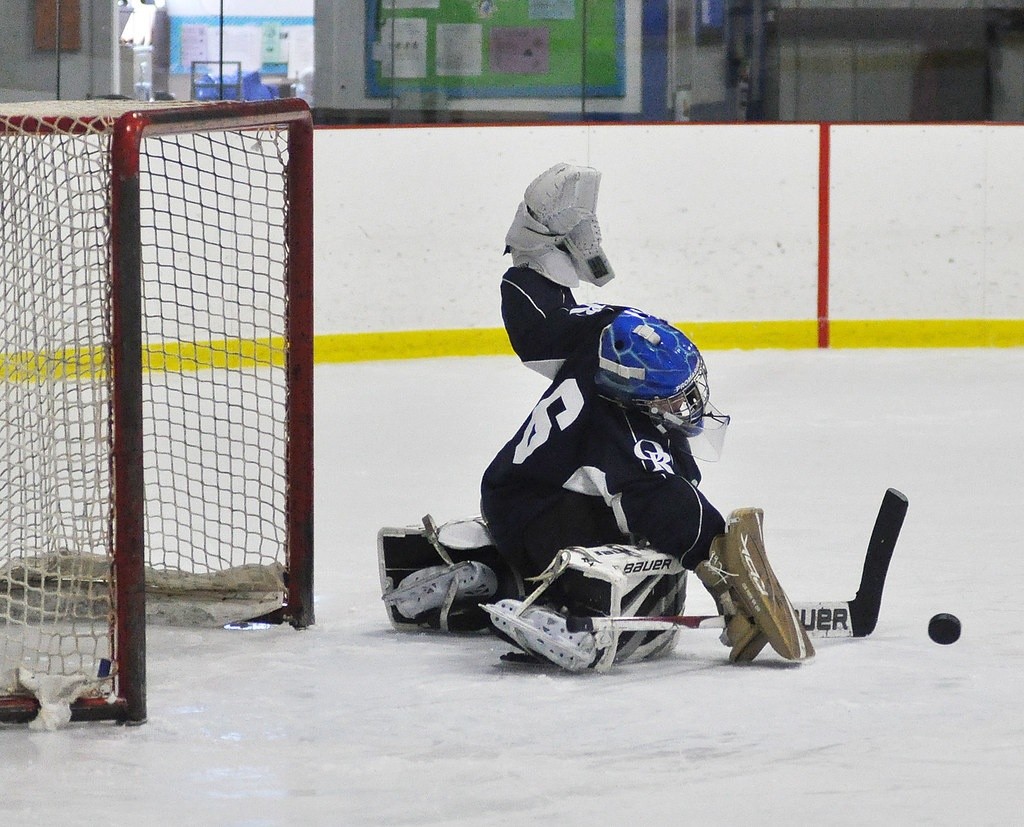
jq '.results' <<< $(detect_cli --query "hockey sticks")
[568,486,909,639]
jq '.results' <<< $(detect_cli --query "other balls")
[928,613,962,645]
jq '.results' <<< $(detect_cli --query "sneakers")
[381,561,497,619]
[477,597,596,672]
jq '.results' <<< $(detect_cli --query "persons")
[377,161,818,675]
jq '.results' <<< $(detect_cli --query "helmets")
[593,309,730,438]
